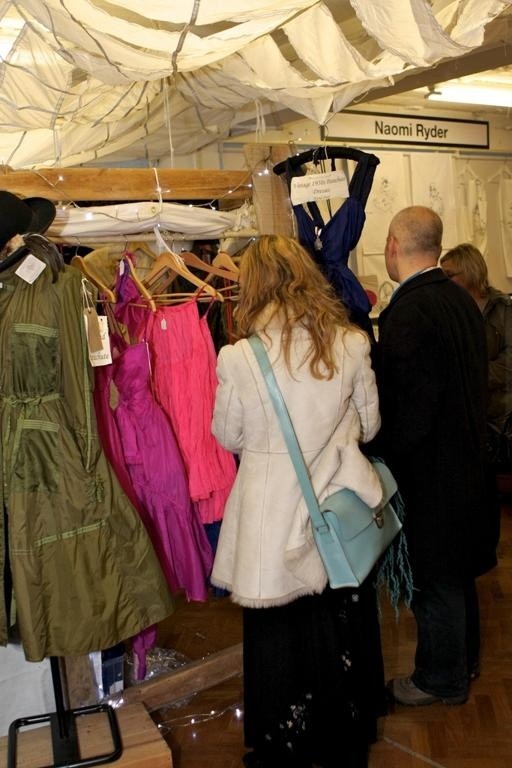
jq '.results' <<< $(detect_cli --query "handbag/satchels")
[310,459,404,592]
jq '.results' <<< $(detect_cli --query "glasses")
[445,273,462,280]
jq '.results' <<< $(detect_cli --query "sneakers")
[385,677,469,707]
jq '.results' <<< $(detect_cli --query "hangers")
[0,231,246,315]
[456,155,512,186]
[269,125,378,175]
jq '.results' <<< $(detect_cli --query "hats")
[0,190,55,273]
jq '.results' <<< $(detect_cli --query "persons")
[0,234,175,662]
[439,244,512,577]
[360,206,501,706]
[209,232,386,768]
